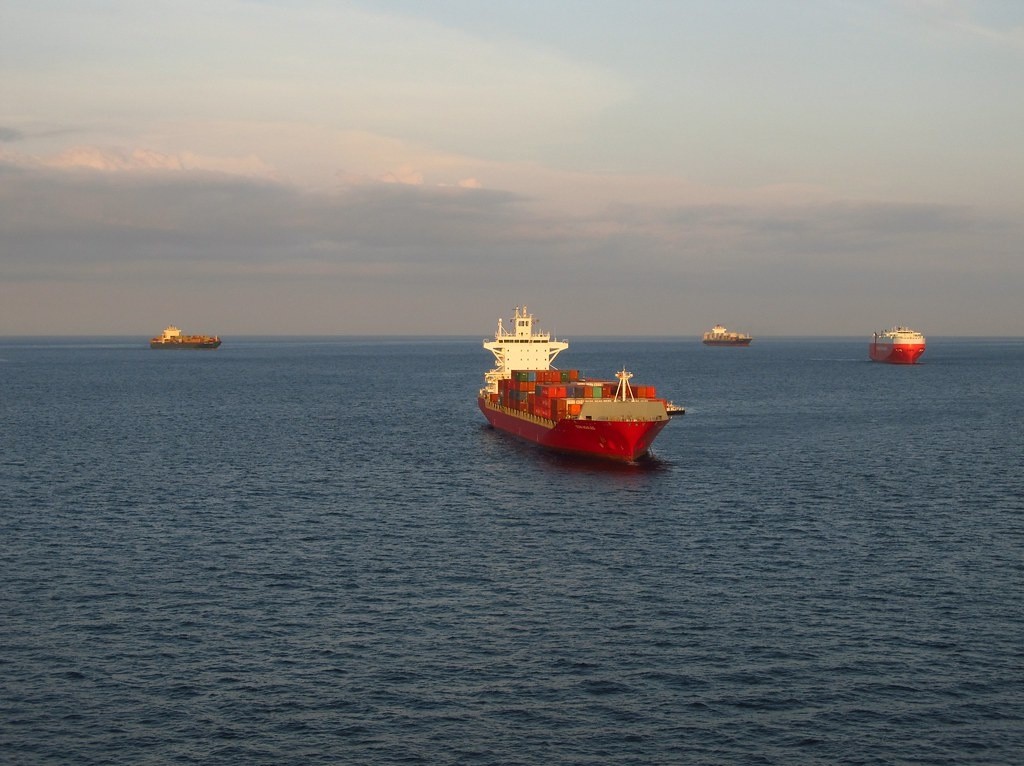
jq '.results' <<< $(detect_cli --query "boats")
[868,325,927,364]
[149,324,223,349]
[700,324,754,347]
[667,399,685,416]
[475,304,675,461]
